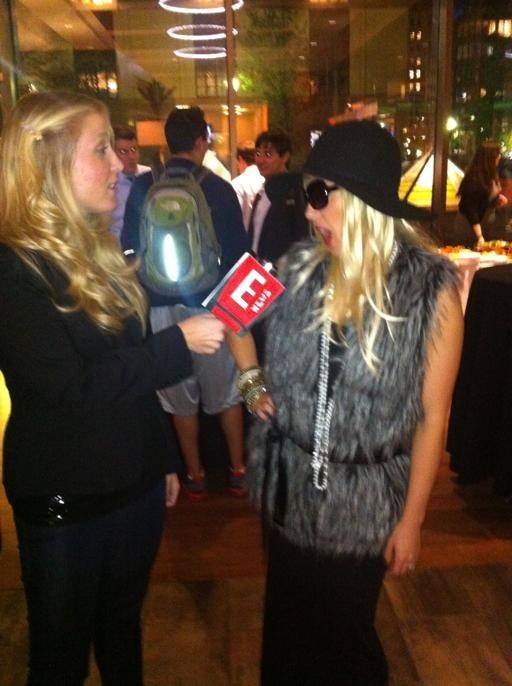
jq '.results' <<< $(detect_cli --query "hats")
[301,117,437,222]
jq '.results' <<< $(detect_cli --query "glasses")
[291,178,338,216]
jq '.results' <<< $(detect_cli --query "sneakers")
[224,467,249,500]
[183,469,208,502]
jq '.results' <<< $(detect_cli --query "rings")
[408,563,416,571]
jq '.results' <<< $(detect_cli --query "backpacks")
[137,159,224,299]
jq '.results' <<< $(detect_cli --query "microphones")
[201,250,286,338]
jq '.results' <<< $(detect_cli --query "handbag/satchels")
[487,216,512,241]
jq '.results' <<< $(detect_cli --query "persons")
[1,91,228,686]
[225,121,465,685]
[203,126,309,262]
[109,127,151,240]
[121,107,247,499]
[453,141,508,252]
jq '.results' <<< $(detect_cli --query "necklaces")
[311,241,397,493]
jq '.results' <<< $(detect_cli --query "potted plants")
[135,78,178,147]
